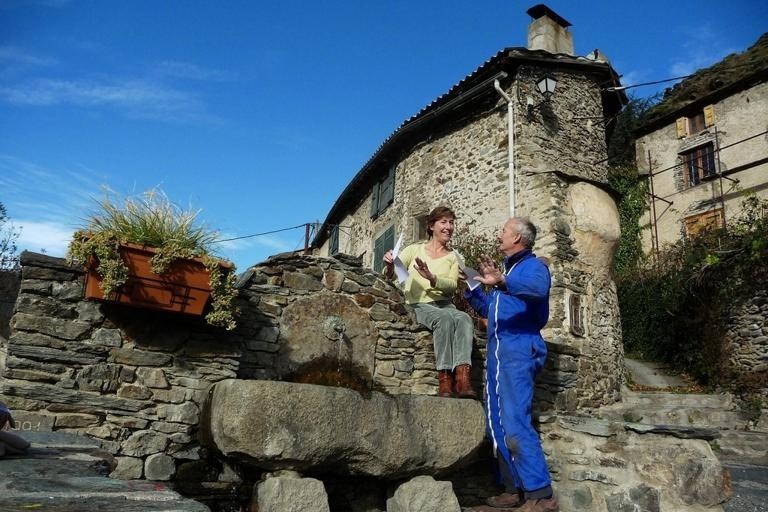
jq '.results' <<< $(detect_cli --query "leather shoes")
[513,499,559,512]
[486,493,520,507]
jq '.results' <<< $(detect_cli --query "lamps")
[527,72,558,114]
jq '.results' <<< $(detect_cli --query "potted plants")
[65,181,242,331]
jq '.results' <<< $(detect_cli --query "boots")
[439,364,477,398]
